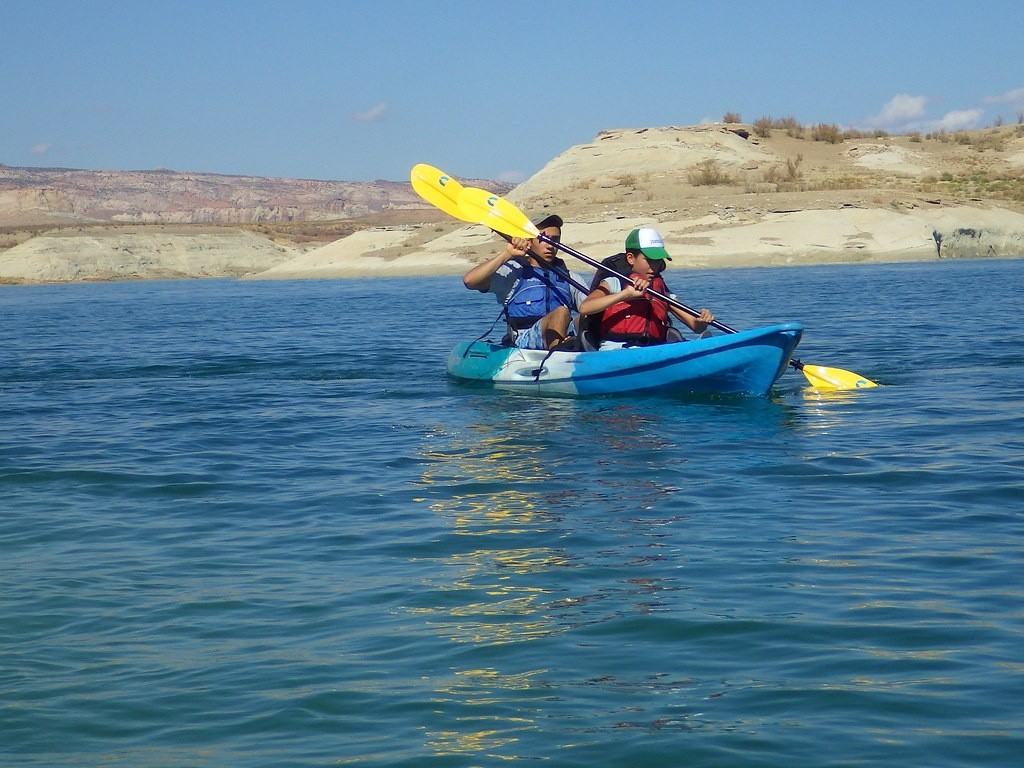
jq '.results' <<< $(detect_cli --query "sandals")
[555,337,578,350]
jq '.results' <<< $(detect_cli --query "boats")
[446,322,805,404]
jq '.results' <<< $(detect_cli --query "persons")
[463,214,589,351]
[579,228,715,355]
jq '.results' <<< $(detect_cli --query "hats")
[533,215,564,229]
[626,227,673,260]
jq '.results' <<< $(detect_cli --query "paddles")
[410,163,590,295]
[459,187,876,392]
